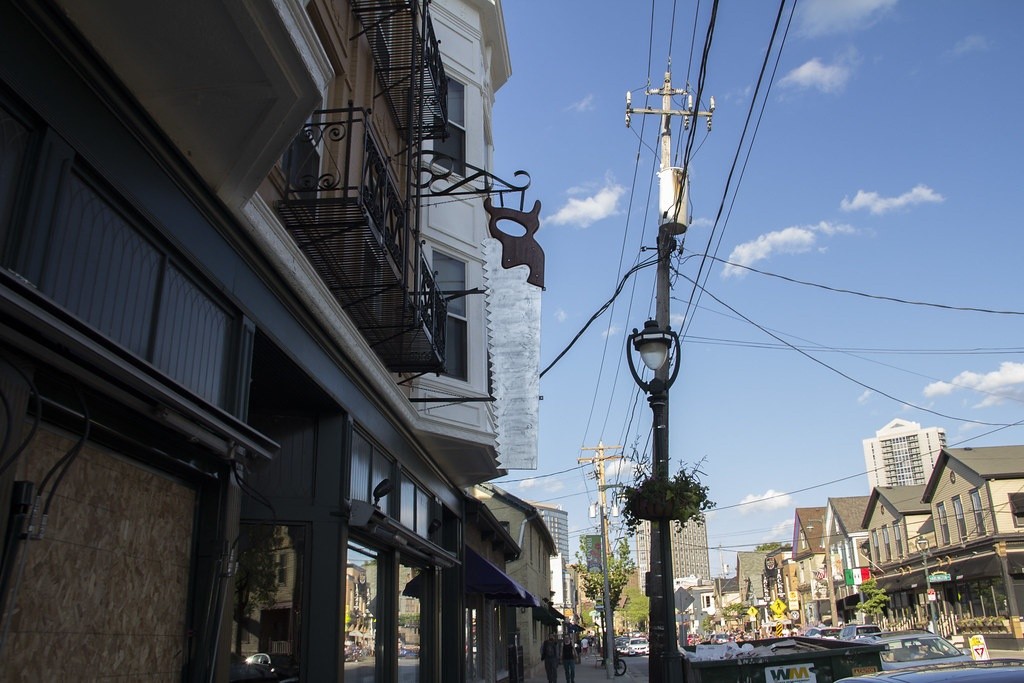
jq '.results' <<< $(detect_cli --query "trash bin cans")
[682,637,892,683]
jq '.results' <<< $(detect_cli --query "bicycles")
[613,649,627,675]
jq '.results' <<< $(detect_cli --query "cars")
[833,657,1024,683]
[849,628,977,671]
[614,631,649,657]
[838,624,882,642]
[687,632,730,646]
[804,627,842,640]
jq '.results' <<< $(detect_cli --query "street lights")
[626,318,683,683]
[914,536,939,635]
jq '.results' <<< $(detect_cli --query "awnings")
[466,544,585,631]
[402,573,423,597]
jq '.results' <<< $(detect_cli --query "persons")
[710,630,806,644]
[910,641,944,659]
[539,632,593,683]
